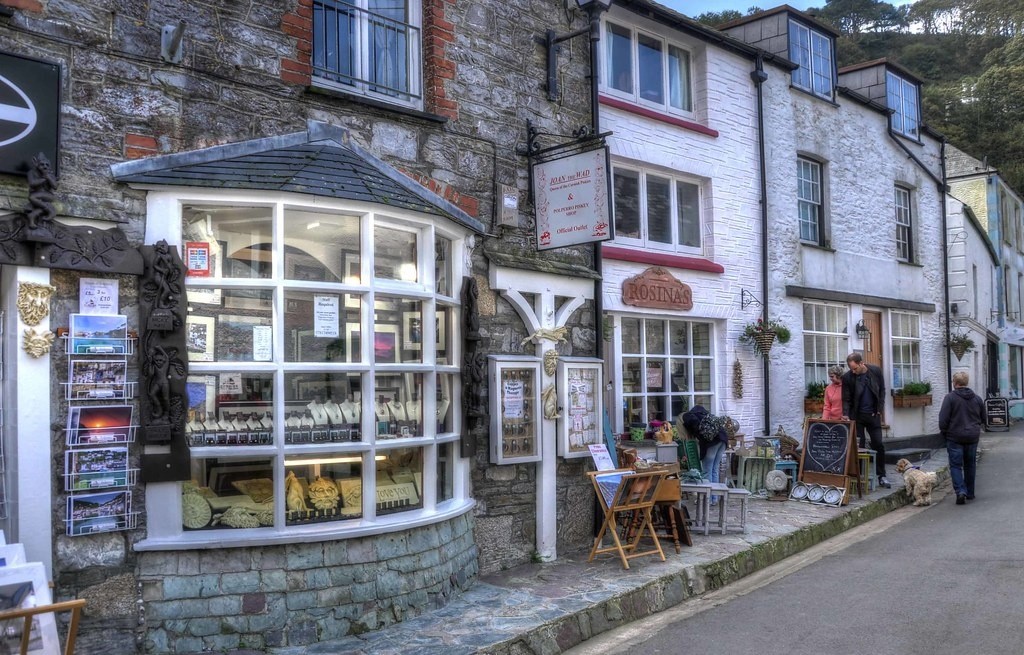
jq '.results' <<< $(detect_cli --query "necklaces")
[391,402,401,409]
[312,400,322,418]
[436,401,444,415]
[409,401,419,412]
[329,403,339,419]
[378,404,384,414]
[347,402,356,417]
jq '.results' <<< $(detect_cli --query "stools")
[850,453,869,494]
[776,474,793,497]
[857,448,877,491]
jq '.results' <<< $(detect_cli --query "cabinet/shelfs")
[486,354,542,465]
[556,357,604,459]
[58,332,141,538]
[620,439,679,464]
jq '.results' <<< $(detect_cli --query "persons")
[675,405,728,507]
[939,371,987,505]
[840,353,892,489]
[822,366,843,421]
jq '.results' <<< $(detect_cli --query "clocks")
[791,481,842,504]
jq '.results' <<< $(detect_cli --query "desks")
[776,462,798,482]
[671,477,750,535]
[620,472,681,554]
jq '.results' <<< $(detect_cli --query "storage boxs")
[737,456,776,499]
[633,461,680,475]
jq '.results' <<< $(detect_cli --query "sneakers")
[879,477,891,488]
[956,494,965,504]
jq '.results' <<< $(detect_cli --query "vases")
[950,343,966,362]
[750,331,776,357]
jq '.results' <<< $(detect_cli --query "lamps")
[856,319,869,339]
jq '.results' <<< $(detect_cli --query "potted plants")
[893,380,933,408]
[804,380,829,414]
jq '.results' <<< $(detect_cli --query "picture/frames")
[207,460,274,497]
[181,235,451,423]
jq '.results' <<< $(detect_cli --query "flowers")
[737,317,790,358]
[943,330,977,353]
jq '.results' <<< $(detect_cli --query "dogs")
[894,458,938,507]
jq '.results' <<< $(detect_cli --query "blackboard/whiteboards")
[799,418,860,475]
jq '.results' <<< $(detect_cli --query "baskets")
[655,422,673,444]
[952,343,968,362]
[753,331,776,357]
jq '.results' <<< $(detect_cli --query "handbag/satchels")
[688,411,722,442]
